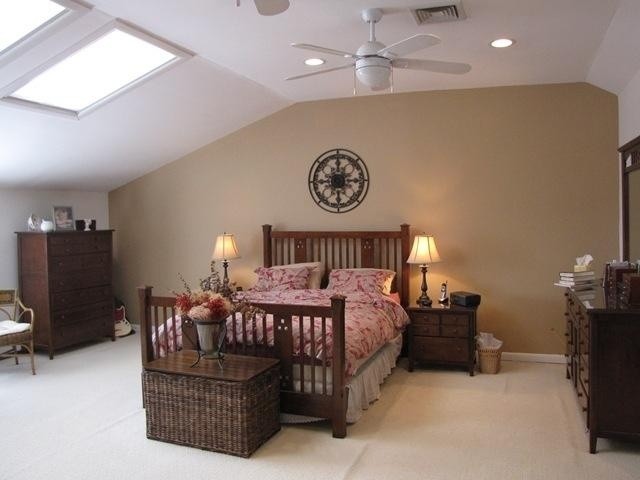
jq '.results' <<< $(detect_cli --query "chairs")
[0,289,35,375]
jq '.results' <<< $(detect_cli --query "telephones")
[437,280,449,306]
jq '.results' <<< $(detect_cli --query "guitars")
[114,294,132,336]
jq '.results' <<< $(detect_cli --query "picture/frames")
[53,206,74,232]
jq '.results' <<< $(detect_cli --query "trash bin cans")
[476,340,503,374]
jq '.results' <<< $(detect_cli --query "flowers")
[168,261,266,324]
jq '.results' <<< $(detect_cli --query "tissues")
[574,253,594,273]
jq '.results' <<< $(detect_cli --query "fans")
[284,8,471,81]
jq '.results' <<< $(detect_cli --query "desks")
[142,350,280,458]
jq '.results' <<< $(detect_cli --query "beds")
[137,223,409,438]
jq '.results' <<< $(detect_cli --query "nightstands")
[406,305,478,376]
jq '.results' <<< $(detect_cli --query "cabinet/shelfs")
[564,278,640,454]
[15,229,116,360]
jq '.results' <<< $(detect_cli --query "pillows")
[252,262,396,296]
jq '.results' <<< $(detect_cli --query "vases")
[197,321,221,352]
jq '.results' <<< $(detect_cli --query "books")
[553,268,598,292]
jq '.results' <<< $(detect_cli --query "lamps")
[406,235,442,306]
[212,232,241,284]
[356,57,394,86]
